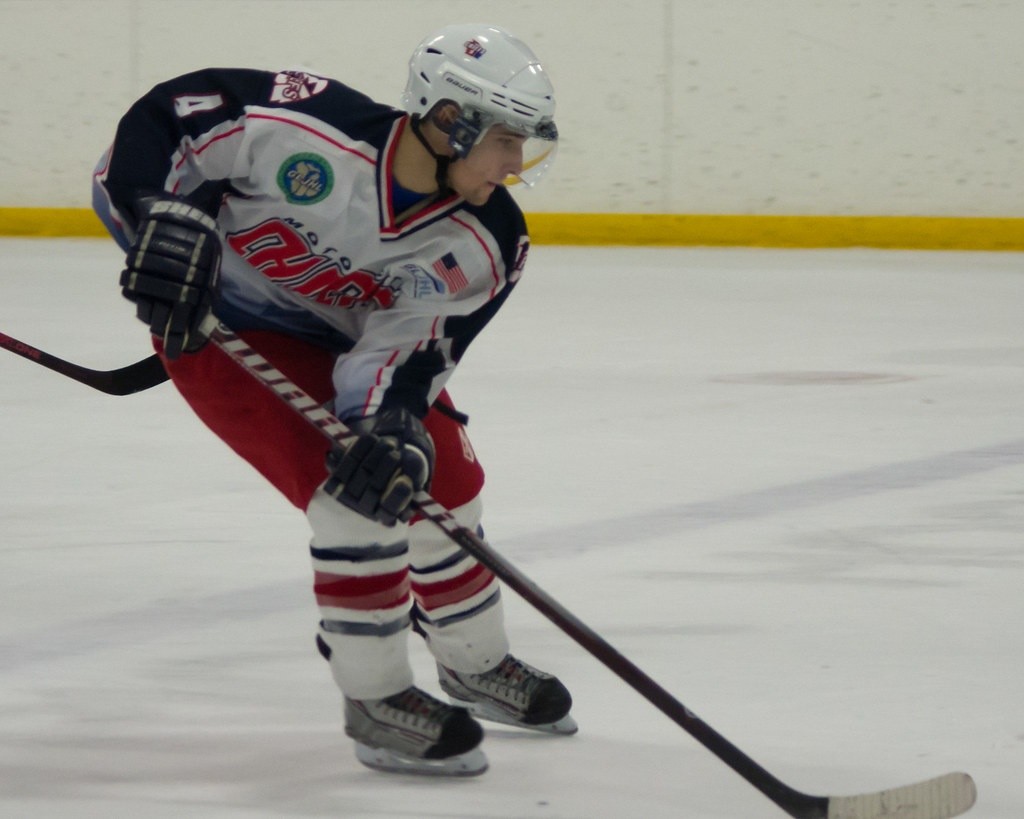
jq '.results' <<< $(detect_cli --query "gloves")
[324,406,436,527]
[118,192,224,361]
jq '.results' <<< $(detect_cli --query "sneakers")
[344,686,489,778]
[438,658,581,735]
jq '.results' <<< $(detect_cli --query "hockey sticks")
[199,315,977,819]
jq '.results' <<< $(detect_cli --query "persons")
[89,24,579,778]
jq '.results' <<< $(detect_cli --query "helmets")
[398,22,556,130]
